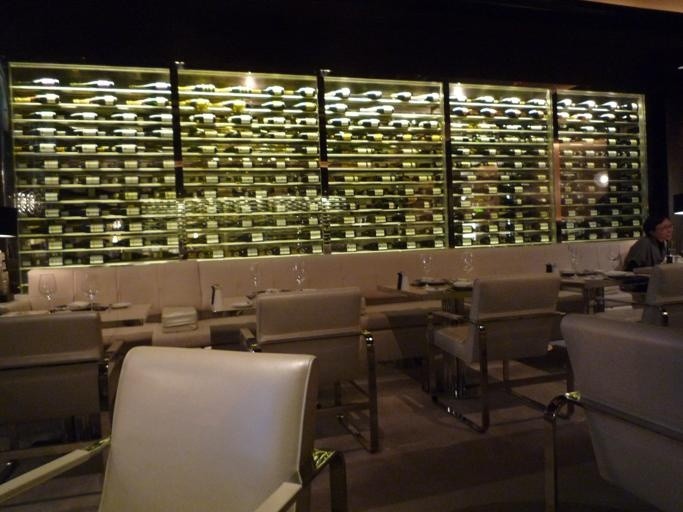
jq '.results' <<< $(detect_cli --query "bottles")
[11,69,178,267]
[551,78,645,241]
[174,74,326,258]
[446,81,553,244]
[319,77,448,251]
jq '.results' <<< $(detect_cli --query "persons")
[619,214,674,306]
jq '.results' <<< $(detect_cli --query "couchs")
[213,262,564,361]
[1,259,211,354]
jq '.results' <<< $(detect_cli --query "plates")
[112,301,132,311]
[231,302,254,307]
[65,300,89,312]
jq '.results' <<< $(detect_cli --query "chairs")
[240,287,380,454]
[643,263,683,326]
[2,347,349,508]
[3,312,125,477]
[425,272,575,434]
[544,312,682,508]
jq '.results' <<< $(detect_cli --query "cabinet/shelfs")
[4,61,649,257]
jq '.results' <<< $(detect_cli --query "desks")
[3,303,152,348]
[207,291,366,410]
[559,268,650,312]
[385,276,587,393]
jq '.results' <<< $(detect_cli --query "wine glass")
[414,253,434,290]
[292,260,310,291]
[248,266,263,295]
[459,252,473,284]
[79,271,103,312]
[38,274,57,316]
[570,252,581,280]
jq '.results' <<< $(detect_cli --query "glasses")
[656,224,674,231]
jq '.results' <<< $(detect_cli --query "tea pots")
[210,282,225,311]
